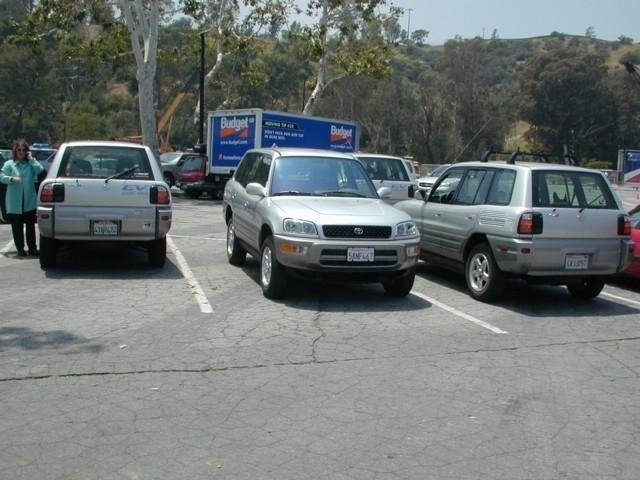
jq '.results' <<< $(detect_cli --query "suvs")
[222,142,422,301]
[392,150,636,302]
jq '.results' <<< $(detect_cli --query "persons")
[1,137,46,259]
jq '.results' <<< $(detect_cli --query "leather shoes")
[28,246,39,256]
[17,248,27,256]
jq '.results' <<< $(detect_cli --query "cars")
[0,141,201,200]
[599,204,640,286]
[36,140,173,267]
[335,152,468,206]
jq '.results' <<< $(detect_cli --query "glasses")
[17,148,26,152]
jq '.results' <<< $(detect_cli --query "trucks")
[173,108,361,199]
